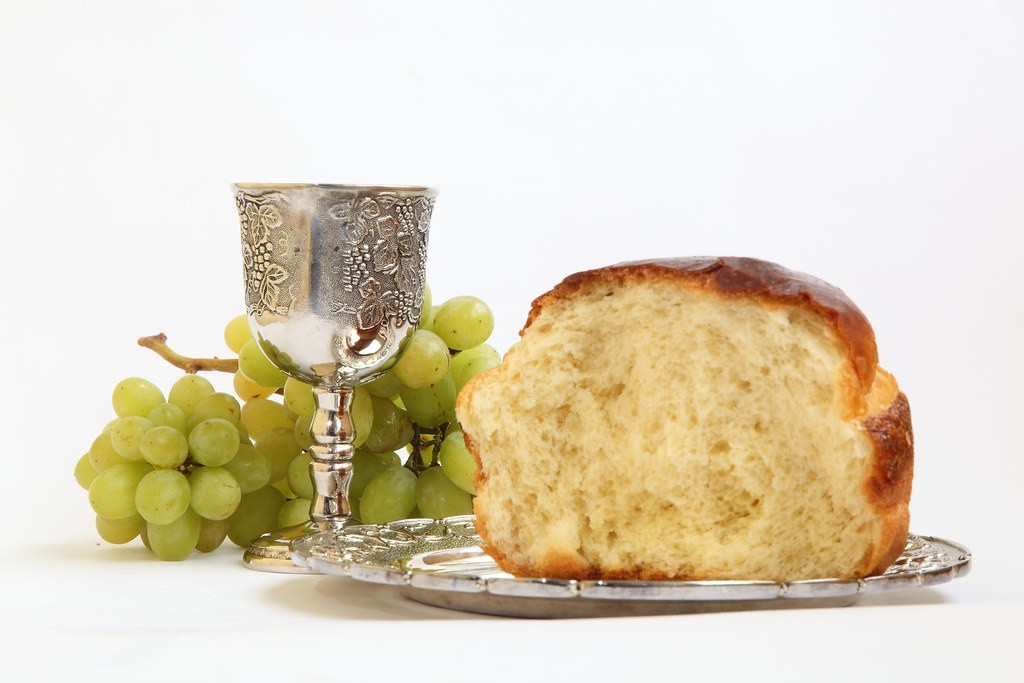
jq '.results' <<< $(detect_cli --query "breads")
[455,254,913,582]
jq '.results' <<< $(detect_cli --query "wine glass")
[232,181,436,574]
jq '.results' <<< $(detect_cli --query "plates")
[293,515,973,606]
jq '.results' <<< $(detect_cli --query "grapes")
[71,278,503,560]
